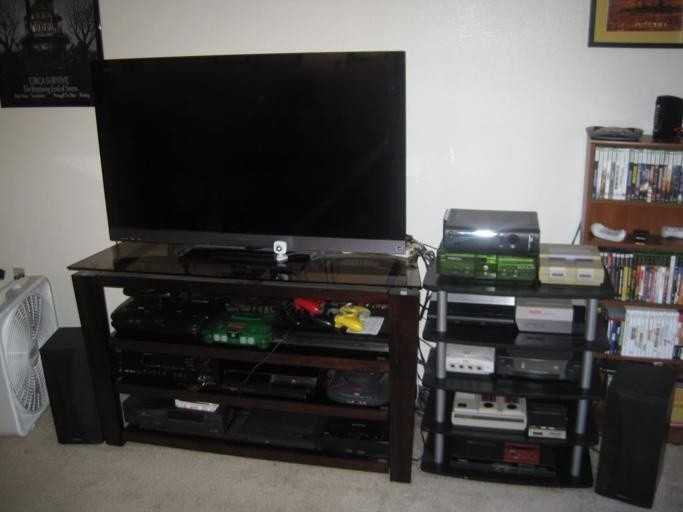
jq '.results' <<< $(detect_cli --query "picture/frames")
[588,0,683,49]
[0,0,103,108]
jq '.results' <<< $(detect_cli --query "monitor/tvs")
[92,52,406,271]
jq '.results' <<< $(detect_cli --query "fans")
[1,276,60,437]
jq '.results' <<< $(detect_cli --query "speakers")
[595,363,676,508]
[39,327,117,444]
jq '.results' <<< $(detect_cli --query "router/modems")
[327,370,390,408]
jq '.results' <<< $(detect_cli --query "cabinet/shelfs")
[419,241,617,493]
[579,134,683,446]
[66,243,422,487]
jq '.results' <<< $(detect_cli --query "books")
[592,146,682,205]
[597,251,683,360]
